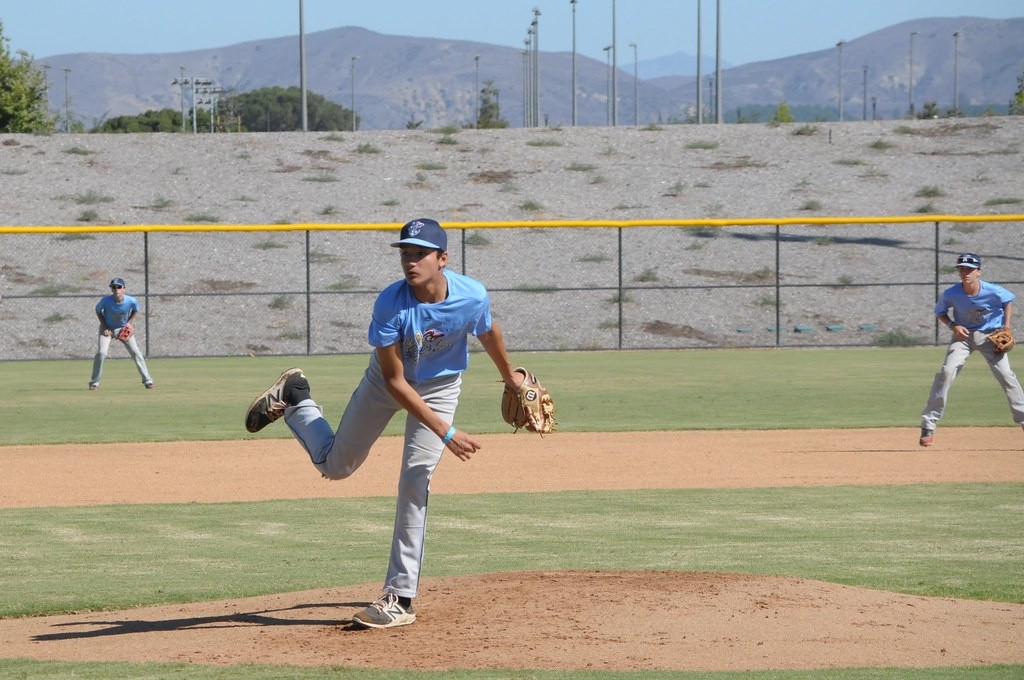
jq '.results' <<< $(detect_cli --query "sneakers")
[352,592,416,628]
[919,428,935,447]
[245,368,310,433]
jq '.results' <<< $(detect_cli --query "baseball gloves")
[119,325,135,342]
[501,366,558,435]
[985,330,1014,353]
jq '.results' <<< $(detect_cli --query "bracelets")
[442,426,456,445]
[947,320,954,328]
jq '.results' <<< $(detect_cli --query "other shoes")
[89,385,97,391]
[146,383,154,388]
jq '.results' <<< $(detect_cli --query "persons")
[89,278,153,390]
[916,254,1024,446]
[245,218,557,628]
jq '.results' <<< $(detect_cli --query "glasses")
[957,258,979,263]
[112,285,124,289]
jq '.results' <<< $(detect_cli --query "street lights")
[909,30,921,121]
[628,42,639,124]
[351,55,361,132]
[603,44,613,126]
[41,62,51,115]
[521,6,542,127]
[180,65,187,133]
[474,55,481,129]
[835,40,846,121]
[62,66,73,134]
[952,31,961,117]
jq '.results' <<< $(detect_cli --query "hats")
[957,254,981,269]
[390,218,447,251]
[108,278,126,287]
